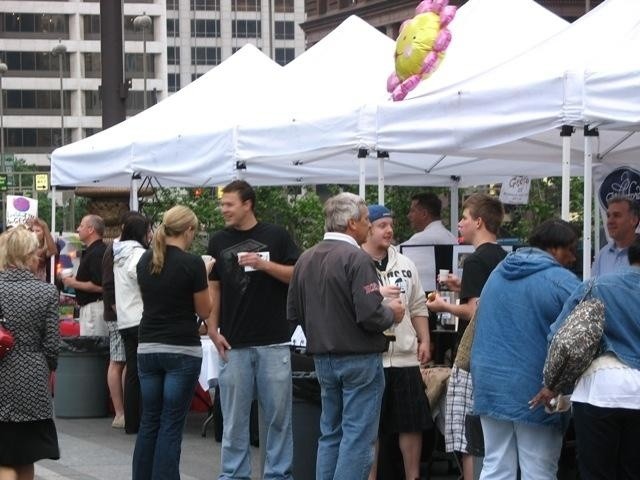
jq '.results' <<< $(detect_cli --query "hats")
[368,204,395,224]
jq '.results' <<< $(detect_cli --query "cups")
[237,252,248,261]
[201,255,212,263]
[440,270,449,281]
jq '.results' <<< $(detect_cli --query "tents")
[49,0,638,287]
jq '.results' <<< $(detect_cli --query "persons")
[359,205,432,480]
[427,193,508,368]
[62,214,108,336]
[101,231,127,427]
[445,298,484,480]
[31,221,57,283]
[287,191,405,480]
[113,214,154,432]
[591,196,640,281]
[394,193,459,296]
[471,219,583,480]
[132,204,216,479]
[0,228,61,480]
[206,180,302,480]
[528,237,640,480]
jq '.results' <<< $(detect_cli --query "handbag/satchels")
[464,401,484,457]
[543,277,605,395]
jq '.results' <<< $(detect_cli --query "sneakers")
[111,416,127,427]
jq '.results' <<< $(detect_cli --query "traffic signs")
[35,174,48,191]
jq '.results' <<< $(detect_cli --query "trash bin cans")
[54,336,111,416]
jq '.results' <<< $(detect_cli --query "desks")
[201,370,465,479]
[430,324,458,365]
